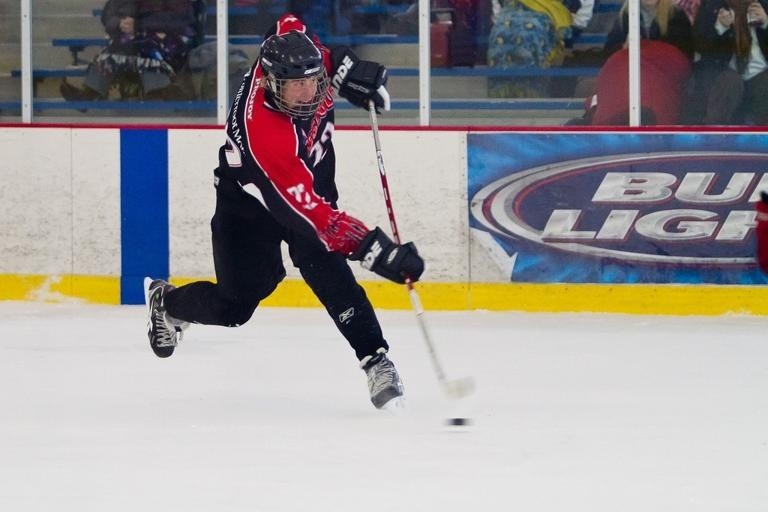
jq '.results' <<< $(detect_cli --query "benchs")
[0,0,627,120]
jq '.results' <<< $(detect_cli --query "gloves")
[349,226,424,284]
[330,46,391,115]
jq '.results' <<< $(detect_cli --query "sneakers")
[359,348,403,408]
[148,280,191,357]
[60,82,88,112]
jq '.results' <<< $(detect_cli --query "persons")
[143,15,425,413]
[60,0,206,105]
[447,0,595,68]
[588,0,768,126]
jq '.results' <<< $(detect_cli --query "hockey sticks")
[366,99,476,399]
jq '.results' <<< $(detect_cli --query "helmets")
[259,30,330,120]
